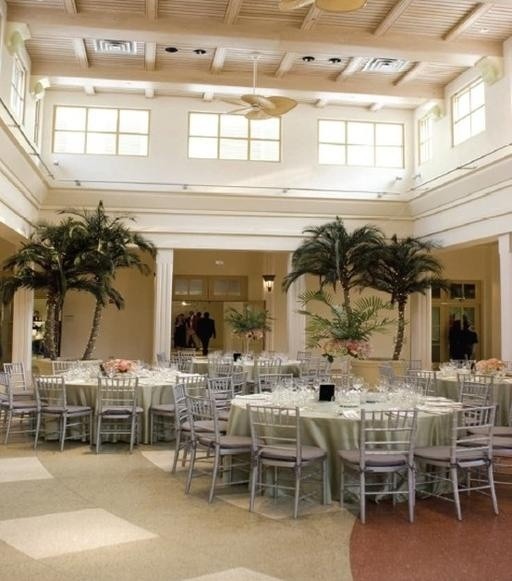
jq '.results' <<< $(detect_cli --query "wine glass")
[270,370,425,410]
[437,358,473,376]
[205,350,289,364]
[65,363,90,380]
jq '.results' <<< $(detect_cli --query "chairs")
[243,402,330,520]
[169,382,222,476]
[18,352,211,454]
[207,376,246,422]
[183,395,261,507]
[412,400,503,521]
[334,409,419,521]
[257,373,291,395]
[176,346,327,379]
[370,348,511,398]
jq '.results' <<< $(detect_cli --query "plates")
[343,396,462,421]
[238,391,278,408]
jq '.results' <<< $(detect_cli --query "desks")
[235,386,465,453]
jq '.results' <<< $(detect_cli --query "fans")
[219,51,298,120]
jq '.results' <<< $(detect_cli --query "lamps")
[263,273,275,292]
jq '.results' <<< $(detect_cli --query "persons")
[448,319,477,360]
[175,311,216,356]
[33,310,39,320]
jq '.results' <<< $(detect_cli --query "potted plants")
[1,198,158,378]
[282,212,445,385]
